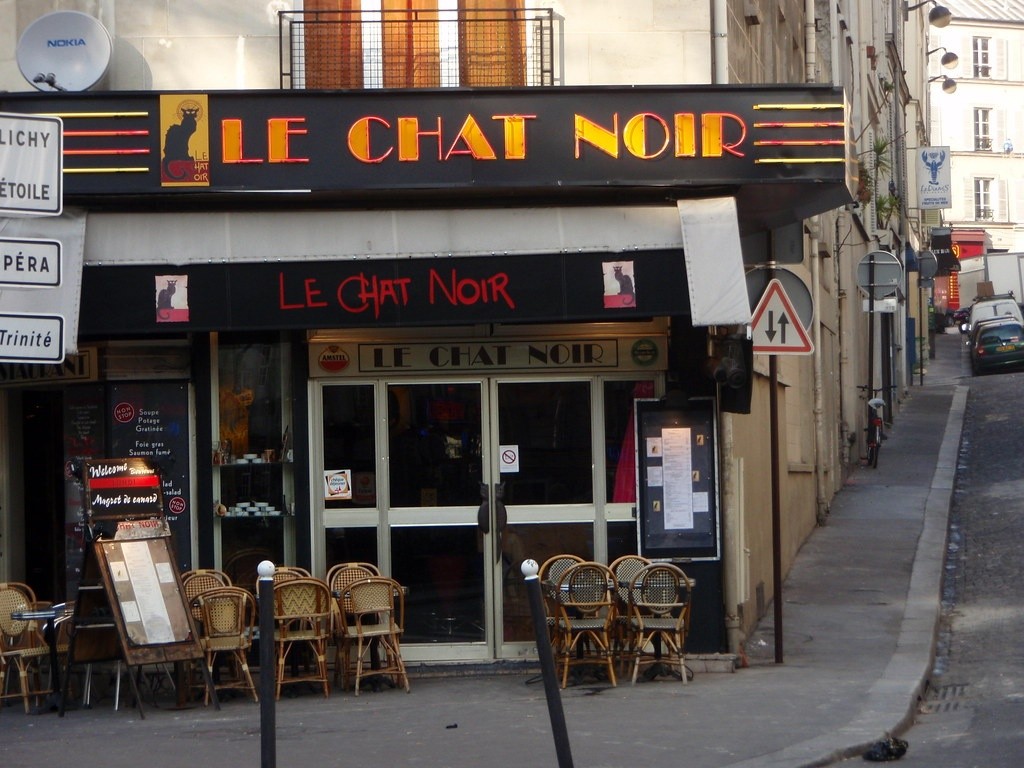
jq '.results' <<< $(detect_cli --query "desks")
[10,605,74,717]
[540,578,615,684]
[330,585,411,694]
[618,579,696,682]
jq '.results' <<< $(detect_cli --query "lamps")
[927,46,959,70]
[928,74,959,93]
[908,0,954,27]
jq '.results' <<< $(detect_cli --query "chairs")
[0,561,411,713]
[606,556,654,677]
[537,555,587,676]
[554,561,618,689]
[627,562,692,686]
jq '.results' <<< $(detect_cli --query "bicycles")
[856,385,897,469]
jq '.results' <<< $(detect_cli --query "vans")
[959,291,1024,337]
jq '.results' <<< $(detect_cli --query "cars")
[954,307,970,320]
[944,310,955,327]
[970,315,1024,376]
